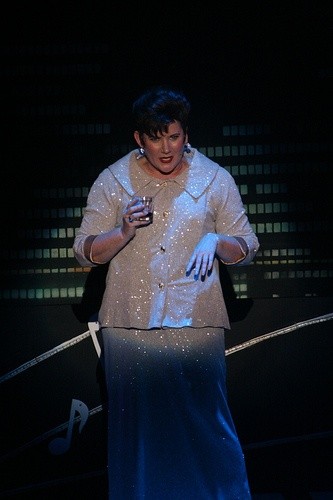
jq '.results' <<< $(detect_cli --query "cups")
[132,196,153,223]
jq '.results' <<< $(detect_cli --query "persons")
[75,90,260,498]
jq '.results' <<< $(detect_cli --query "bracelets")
[208,232,219,246]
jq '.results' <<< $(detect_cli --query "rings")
[127,216,133,222]
